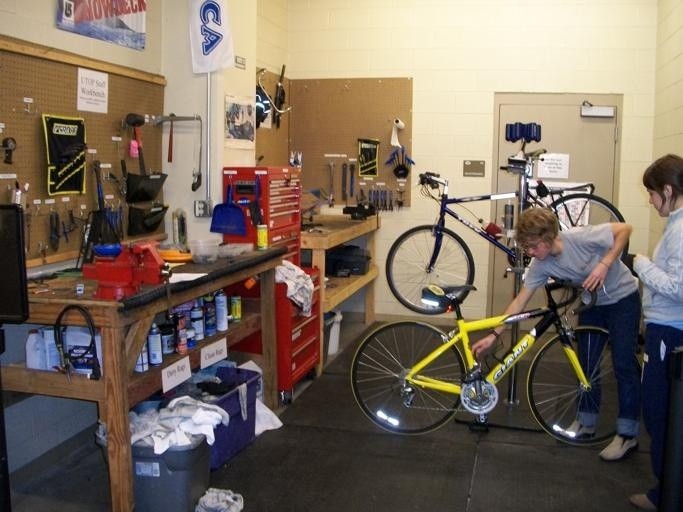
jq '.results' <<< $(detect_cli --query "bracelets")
[488,328,502,338]
[597,260,609,266]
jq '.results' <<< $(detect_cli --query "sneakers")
[598,434,639,461]
[557,419,595,443]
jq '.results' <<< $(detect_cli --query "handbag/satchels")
[195,486,244,512]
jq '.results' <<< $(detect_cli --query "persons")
[469,206,644,462]
[628,153,682,511]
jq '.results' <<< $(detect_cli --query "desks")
[0,243,288,512]
[300,214,382,377]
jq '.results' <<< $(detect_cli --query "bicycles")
[350,277,641,447]
[386,148,629,315]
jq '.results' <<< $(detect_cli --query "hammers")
[168,113,176,162]
[126,113,147,176]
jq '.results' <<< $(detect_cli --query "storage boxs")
[93,366,261,512]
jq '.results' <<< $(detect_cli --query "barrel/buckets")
[324,312,337,364]
[328,310,344,357]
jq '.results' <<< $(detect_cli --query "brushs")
[249,175,262,225]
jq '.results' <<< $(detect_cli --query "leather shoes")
[629,493,655,511]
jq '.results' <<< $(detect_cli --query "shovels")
[91,160,121,246]
[209,185,249,237]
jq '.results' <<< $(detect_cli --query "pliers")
[110,160,128,196]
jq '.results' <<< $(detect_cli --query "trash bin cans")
[93,399,214,508]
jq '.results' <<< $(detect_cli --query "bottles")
[478,218,502,239]
[133,288,244,377]
